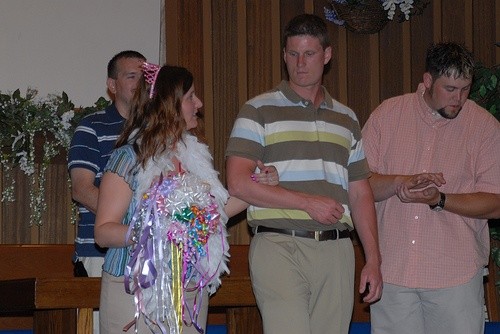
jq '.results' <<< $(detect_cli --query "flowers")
[323,0,414,26]
[1,87,112,226]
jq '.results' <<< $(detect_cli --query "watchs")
[429,192,446,212]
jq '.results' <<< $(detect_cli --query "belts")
[258,225,350,242]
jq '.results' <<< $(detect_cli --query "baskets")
[328,1,396,34]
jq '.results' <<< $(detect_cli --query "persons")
[226,13,381,334]
[362,39,500,334]
[95,64,279,334]
[67,51,148,334]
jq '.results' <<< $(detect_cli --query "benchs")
[0,277,264,334]
